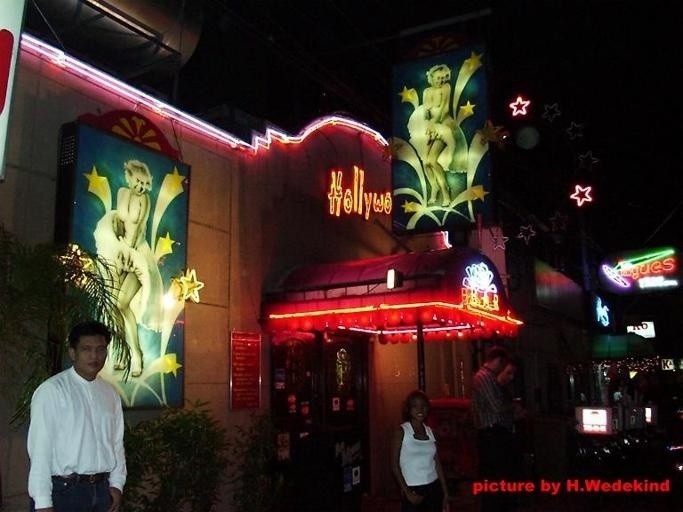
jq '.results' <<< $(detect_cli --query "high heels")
[114,344,144,377]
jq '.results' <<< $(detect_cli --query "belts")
[58,473,108,484]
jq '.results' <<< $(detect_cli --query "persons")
[94,160,164,377]
[407,64,468,207]
[27,322,127,512]
[467,346,521,512]
[391,390,449,512]
[496,360,527,512]
